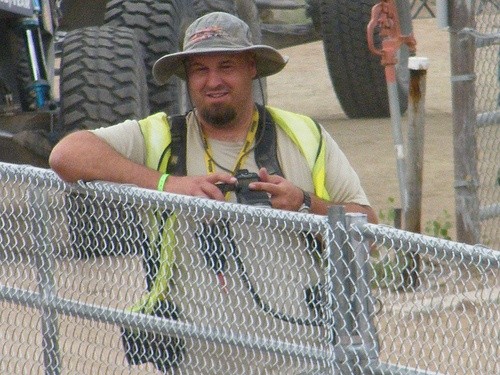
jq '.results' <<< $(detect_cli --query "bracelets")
[157,173,168,192]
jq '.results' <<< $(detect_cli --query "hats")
[151,11,290,85]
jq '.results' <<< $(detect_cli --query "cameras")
[213,169,272,209]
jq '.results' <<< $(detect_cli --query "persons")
[48,12,378,375]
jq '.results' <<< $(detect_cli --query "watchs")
[297,188,312,215]
